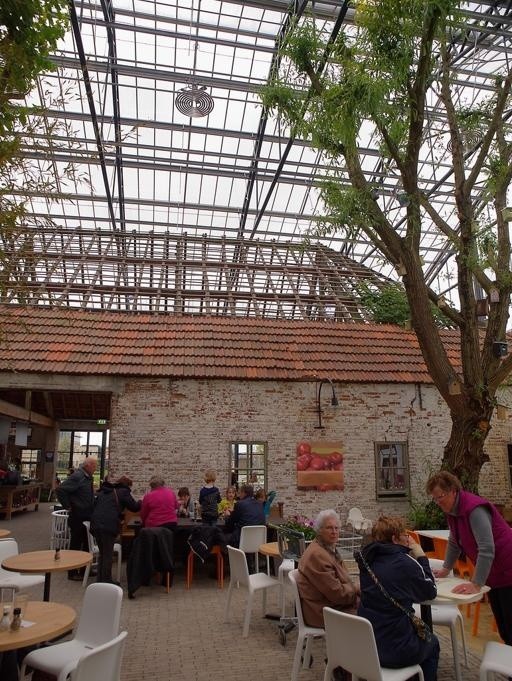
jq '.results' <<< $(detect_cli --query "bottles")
[10,607,21,632]
[55,547,61,562]
[0,605,11,631]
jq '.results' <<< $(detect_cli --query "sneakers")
[80,569,98,576]
[324,658,343,681]
[68,573,84,581]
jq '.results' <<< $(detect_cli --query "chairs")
[289,560,511,681]
[20,583,130,681]
[0,537,45,604]
[222,545,285,637]
[237,526,274,577]
[81,521,125,587]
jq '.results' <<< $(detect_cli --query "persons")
[352,513,440,681]
[425,471,511,647]
[55,472,61,486]
[55,455,97,581]
[199,469,222,526]
[175,487,196,517]
[89,475,144,586]
[222,483,265,574]
[140,475,180,587]
[382,465,403,490]
[253,488,276,521]
[295,509,361,681]
[217,484,238,518]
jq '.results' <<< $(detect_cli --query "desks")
[0,601,77,653]
[416,578,484,606]
[0,549,94,601]
[126,513,243,530]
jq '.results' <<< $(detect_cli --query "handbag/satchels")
[413,616,434,642]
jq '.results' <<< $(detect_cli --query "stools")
[185,544,224,588]
